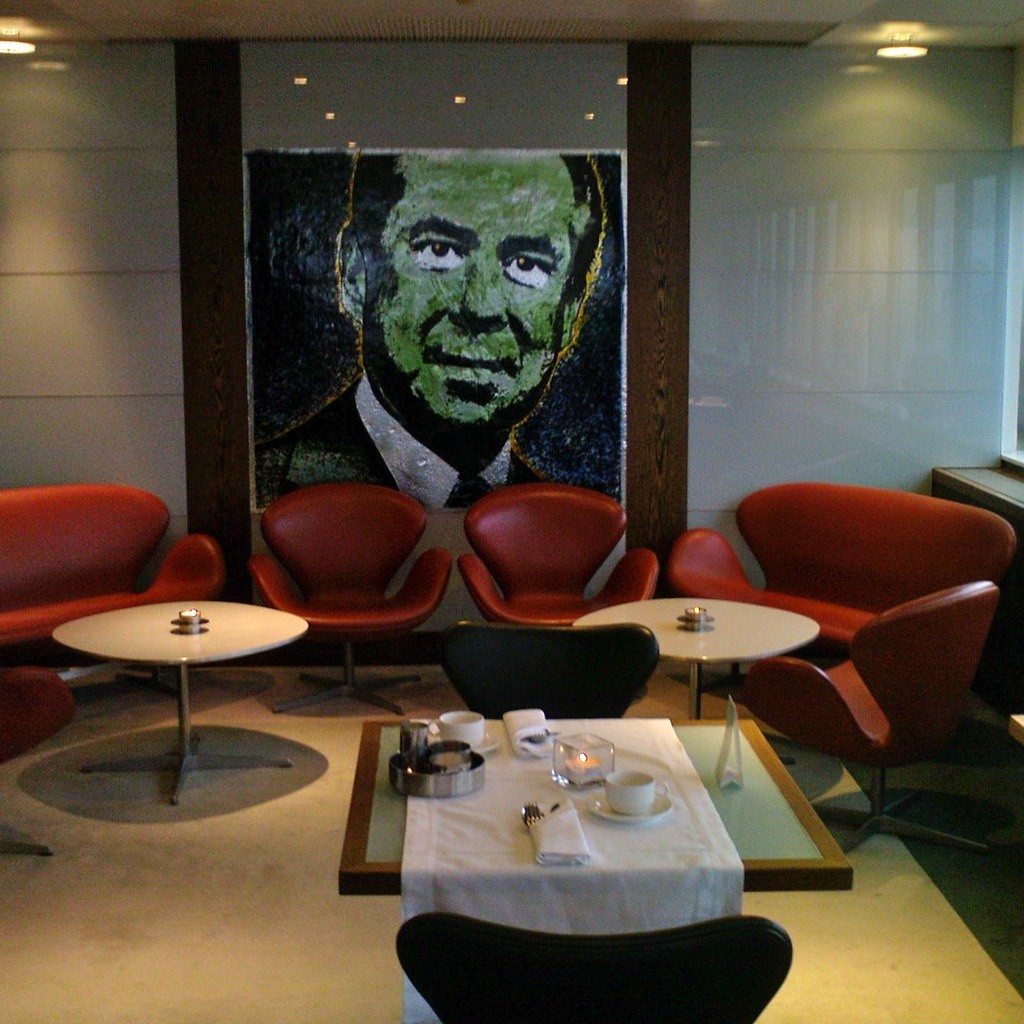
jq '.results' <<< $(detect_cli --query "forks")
[524,799,543,830]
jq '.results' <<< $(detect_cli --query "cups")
[604,770,669,816]
[429,710,485,751]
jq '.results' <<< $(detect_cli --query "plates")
[425,730,497,755]
[587,789,672,825]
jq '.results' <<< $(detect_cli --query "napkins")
[504,709,553,759]
[528,799,592,865]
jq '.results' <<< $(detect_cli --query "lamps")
[876,33,931,59]
[0,29,35,55]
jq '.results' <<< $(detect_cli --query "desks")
[51,603,308,805]
[339,717,854,896]
[573,596,821,721]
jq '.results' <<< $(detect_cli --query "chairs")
[667,483,1015,857]
[0,476,226,645]
[247,483,452,717]
[0,664,74,857]
[458,482,659,625]
[437,619,658,720]
[395,912,794,1024]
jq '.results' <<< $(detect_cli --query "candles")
[677,606,712,631]
[171,608,209,631]
[553,734,616,789]
